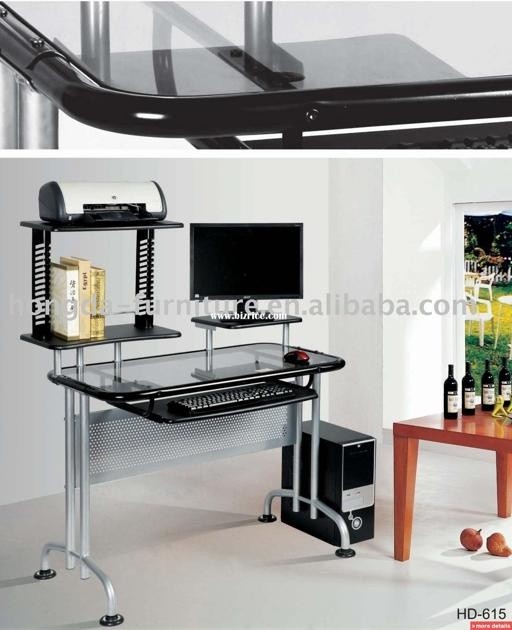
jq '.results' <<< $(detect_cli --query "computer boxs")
[280,418,377,546]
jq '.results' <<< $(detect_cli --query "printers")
[38,181,169,225]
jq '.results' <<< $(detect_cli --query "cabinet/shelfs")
[20,219,184,376]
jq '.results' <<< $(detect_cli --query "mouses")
[284,350,311,363]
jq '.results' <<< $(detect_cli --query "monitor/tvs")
[188,223,306,323]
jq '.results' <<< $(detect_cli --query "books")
[50,256,106,341]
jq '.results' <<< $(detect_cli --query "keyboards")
[168,385,294,411]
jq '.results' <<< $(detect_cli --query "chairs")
[465,271,495,346]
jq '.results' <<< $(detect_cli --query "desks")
[392,403,511,561]
[494,295,512,348]
[34,310,355,626]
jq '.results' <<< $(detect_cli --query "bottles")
[481,359,495,411]
[443,363,459,420]
[461,361,477,416]
[498,356,511,407]
